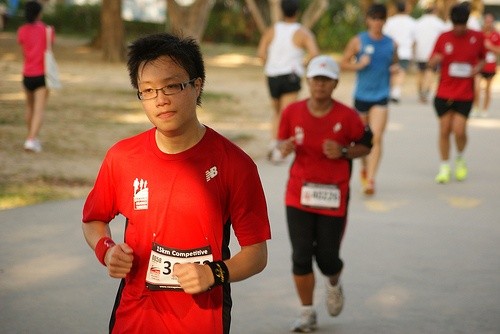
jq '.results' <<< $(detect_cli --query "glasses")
[137,78,197,100]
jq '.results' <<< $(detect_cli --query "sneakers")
[291,310,319,332]
[436,165,450,184]
[455,156,467,181]
[325,281,344,316]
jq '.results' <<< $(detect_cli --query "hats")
[307,55,340,80]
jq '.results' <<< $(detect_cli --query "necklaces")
[309,99,332,111]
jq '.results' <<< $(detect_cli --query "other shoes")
[365,180,375,196]
[361,171,368,182]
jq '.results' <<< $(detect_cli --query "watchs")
[341,145,347,158]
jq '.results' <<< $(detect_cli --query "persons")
[258,0,319,166]
[17,2,55,152]
[276,54,373,333]
[382,1,500,118]
[82,26,272,334]
[339,3,399,193]
[428,5,488,183]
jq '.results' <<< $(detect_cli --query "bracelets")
[203,261,230,292]
